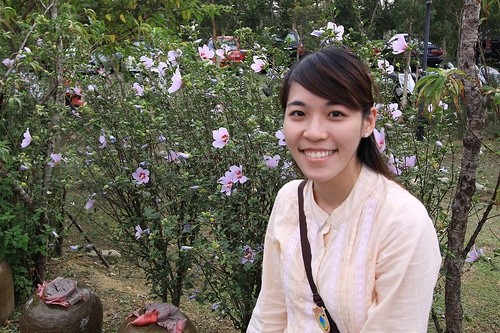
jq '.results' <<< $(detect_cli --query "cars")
[398,39,443,65]
[252,28,305,70]
[208,36,247,67]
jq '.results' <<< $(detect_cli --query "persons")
[246,47,442,333]
